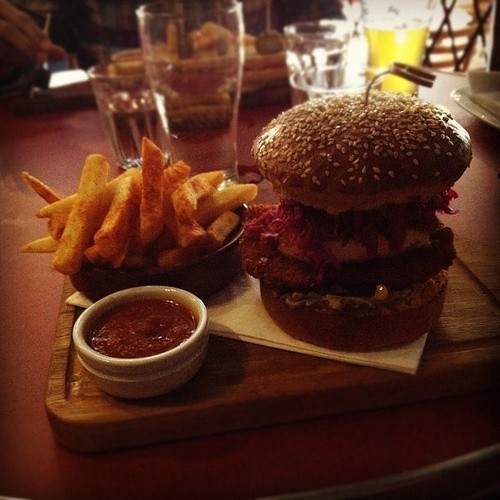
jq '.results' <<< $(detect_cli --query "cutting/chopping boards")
[45,234,500,453]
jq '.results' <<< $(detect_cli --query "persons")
[0,0,346,64]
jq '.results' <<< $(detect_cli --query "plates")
[450,84,500,129]
[70,203,247,297]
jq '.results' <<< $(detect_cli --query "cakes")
[106,22,288,86]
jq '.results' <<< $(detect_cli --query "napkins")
[468,71,500,117]
[63,267,430,374]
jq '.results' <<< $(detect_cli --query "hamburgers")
[161,72,233,130]
[241,93,472,351]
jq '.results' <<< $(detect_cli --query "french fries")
[21,137,258,275]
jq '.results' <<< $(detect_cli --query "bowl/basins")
[74,284,211,401]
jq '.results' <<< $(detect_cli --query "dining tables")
[0,69,500,500]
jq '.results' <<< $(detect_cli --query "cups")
[135,0,246,185]
[288,66,383,107]
[283,20,356,106]
[88,57,169,173]
[362,0,430,98]
